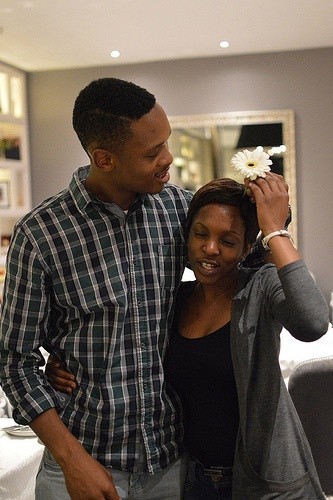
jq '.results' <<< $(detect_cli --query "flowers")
[230,146,273,197]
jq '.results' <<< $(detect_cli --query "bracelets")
[261,229,295,254]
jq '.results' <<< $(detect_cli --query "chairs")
[288,356,333,497]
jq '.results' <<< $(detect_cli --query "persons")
[0,78,289,500]
[44,172,329,500]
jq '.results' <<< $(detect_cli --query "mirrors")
[166,109,298,254]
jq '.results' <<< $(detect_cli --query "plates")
[6,426,37,436]
